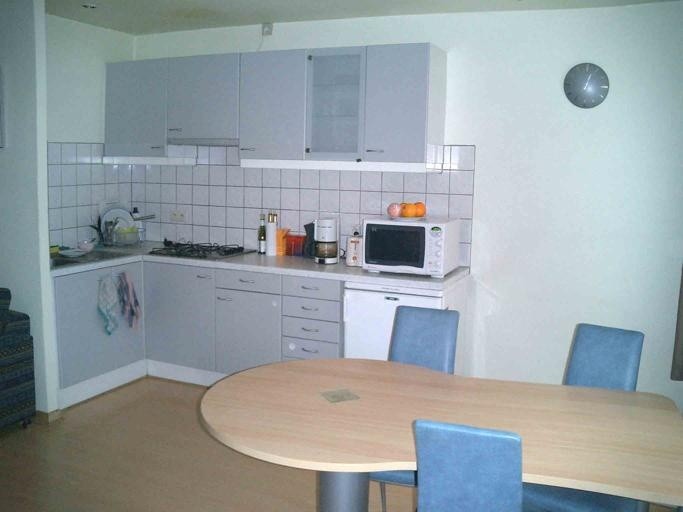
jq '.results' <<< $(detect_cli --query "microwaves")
[362,215,459,277]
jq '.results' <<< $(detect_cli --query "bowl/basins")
[49,245,58,258]
[286,235,307,255]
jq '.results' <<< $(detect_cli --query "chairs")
[411,417,522,511]
[1,286,36,434]
[366,305,460,512]
[520,322,644,511]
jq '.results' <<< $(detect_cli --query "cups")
[276,228,286,255]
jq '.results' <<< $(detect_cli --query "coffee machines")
[313,218,338,264]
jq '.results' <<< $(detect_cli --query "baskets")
[99,228,146,248]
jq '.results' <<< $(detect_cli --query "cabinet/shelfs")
[240,49,305,170]
[343,271,471,376]
[365,41,447,174]
[142,259,213,387]
[166,53,239,147]
[213,267,281,384]
[281,274,343,361]
[101,57,198,167]
[53,259,147,411]
[305,46,365,171]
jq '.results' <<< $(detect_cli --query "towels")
[96,273,120,339]
[119,270,143,333]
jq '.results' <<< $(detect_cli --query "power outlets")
[168,211,184,222]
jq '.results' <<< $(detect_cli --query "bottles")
[79,239,93,253]
[267,212,277,224]
[256,213,266,254]
[344,231,362,266]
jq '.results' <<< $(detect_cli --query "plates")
[57,250,85,258]
[387,214,425,221]
[100,208,135,233]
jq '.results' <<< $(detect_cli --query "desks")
[196,357,683,511]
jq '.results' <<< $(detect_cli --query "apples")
[387,202,401,217]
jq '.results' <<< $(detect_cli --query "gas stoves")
[149,241,256,261]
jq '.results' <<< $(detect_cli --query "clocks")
[561,62,610,110]
